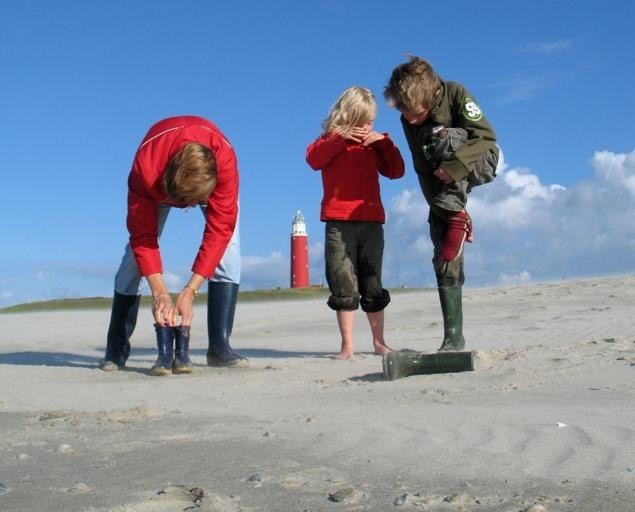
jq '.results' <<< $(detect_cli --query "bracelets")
[184,285,199,297]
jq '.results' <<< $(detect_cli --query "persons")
[382,52,500,353]
[98,115,250,372]
[305,86,402,362]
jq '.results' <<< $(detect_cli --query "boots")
[173,325,191,374]
[383,349,479,381]
[206,281,249,367]
[104,292,141,372]
[150,323,174,376]
[439,286,465,353]
